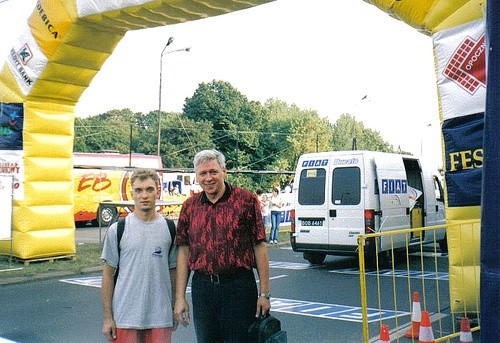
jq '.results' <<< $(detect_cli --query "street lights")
[158,36,191,157]
[352,94,368,149]
[421,123,432,157]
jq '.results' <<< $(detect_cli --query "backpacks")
[247,313,287,343]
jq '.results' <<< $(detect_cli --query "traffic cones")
[378,324,391,343]
[459,317,474,343]
[404,292,436,343]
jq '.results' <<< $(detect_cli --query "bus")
[73,166,204,228]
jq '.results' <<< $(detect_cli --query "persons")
[261,195,269,235]
[256,188,263,201]
[269,188,283,244]
[100,168,179,343]
[174,148,271,343]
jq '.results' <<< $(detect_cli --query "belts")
[195,268,252,284]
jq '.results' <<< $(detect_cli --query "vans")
[290,149,448,264]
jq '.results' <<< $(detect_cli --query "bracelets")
[259,293,270,300]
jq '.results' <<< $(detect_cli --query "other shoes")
[269,239,278,244]
